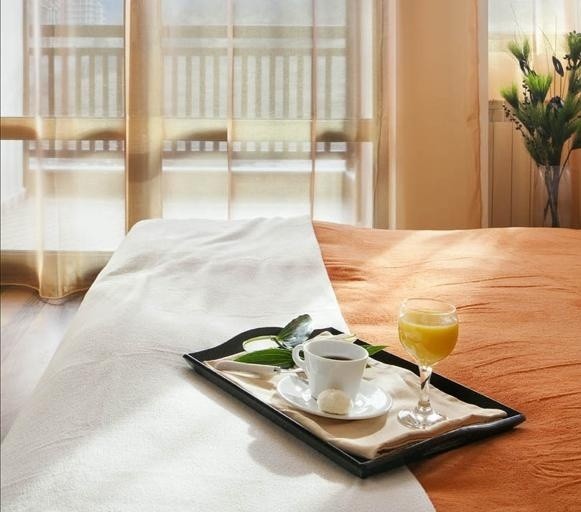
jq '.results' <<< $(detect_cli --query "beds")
[1,217,581,512]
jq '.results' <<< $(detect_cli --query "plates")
[278,369,392,420]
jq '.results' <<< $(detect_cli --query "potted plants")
[498,4,581,227]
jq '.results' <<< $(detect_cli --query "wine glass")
[397,298,458,430]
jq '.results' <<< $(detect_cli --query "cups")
[292,339,369,405]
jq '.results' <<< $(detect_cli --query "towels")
[203,352,507,460]
